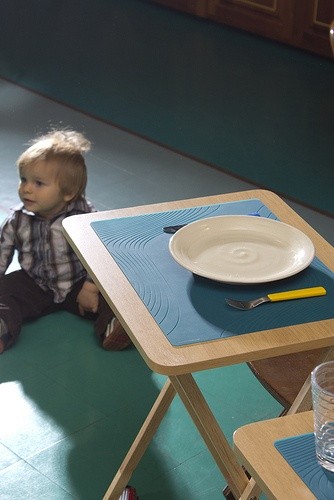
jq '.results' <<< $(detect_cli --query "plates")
[168,214,315,283]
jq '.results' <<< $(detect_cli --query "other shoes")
[102,317,130,351]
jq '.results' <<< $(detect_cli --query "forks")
[225,286,326,309]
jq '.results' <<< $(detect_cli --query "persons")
[0,131,131,355]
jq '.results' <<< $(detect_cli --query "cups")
[310,360,334,472]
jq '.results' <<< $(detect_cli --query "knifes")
[161,212,261,233]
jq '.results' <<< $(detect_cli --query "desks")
[233,406,334,500]
[61,190,334,500]
